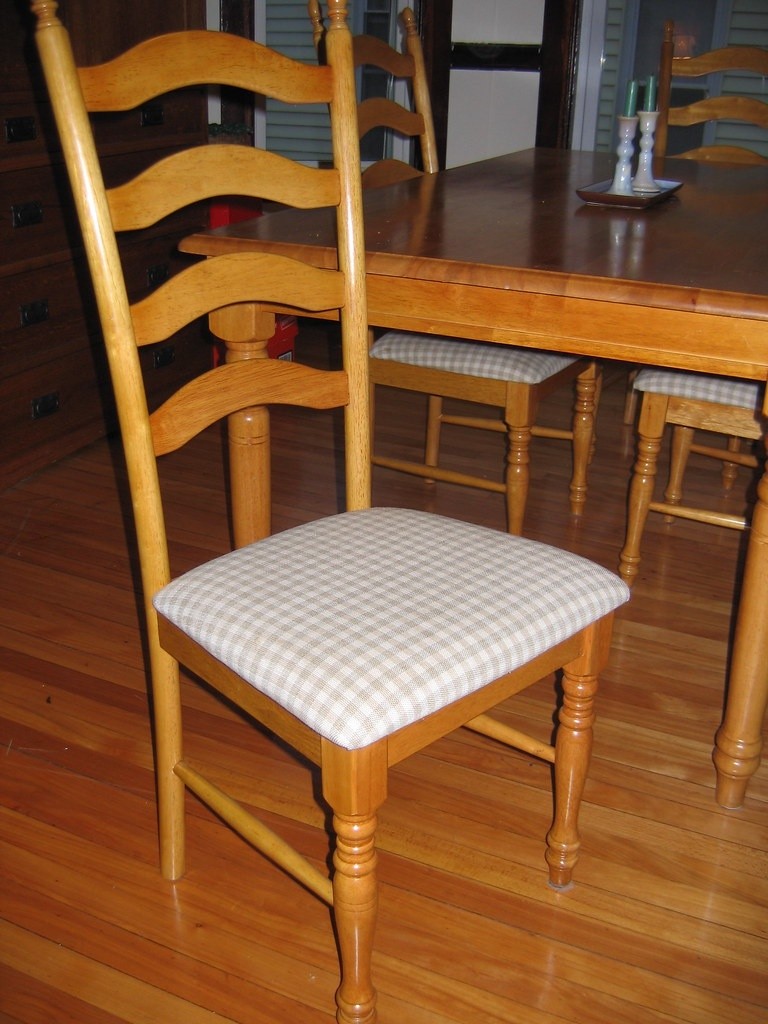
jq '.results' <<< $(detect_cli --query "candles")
[644,72,657,112]
[624,77,639,117]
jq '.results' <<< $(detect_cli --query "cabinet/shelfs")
[0,0,212,491]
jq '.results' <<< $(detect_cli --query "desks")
[180,149,768,808]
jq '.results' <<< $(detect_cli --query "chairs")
[308,0,606,536]
[30,0,632,1023]
[618,16,767,589]
